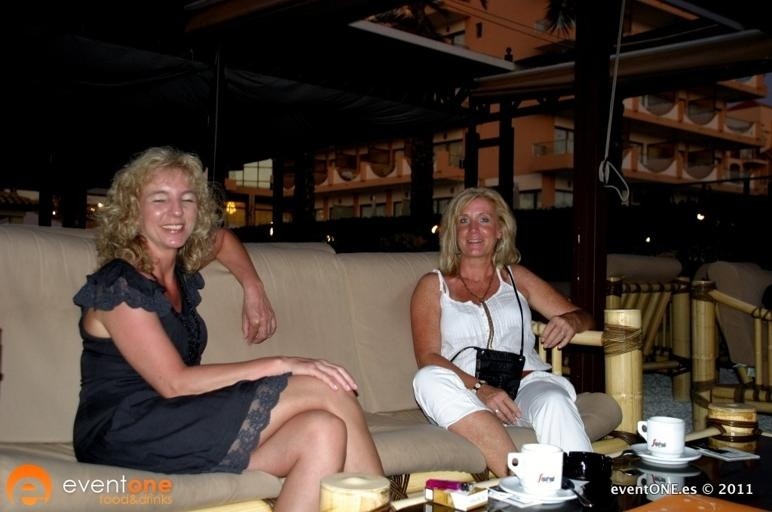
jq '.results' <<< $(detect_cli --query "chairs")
[606,253,772,431]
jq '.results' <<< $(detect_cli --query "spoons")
[562,477,594,509]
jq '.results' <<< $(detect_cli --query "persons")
[72,147,388,512]
[409,187,595,481]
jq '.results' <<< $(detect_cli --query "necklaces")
[458,261,498,346]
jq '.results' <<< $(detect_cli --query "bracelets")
[470,379,487,395]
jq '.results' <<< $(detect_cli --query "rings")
[495,409,499,413]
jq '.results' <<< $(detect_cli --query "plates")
[630,441,704,469]
[498,475,586,504]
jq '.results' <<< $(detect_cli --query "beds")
[319,403,772,512]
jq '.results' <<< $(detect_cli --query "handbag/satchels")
[474,348,525,400]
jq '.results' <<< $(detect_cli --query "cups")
[636,415,686,458]
[506,442,564,497]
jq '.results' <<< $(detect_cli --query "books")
[698,445,760,463]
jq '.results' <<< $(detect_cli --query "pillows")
[708,261,772,367]
[607,255,682,282]
[0,222,440,442]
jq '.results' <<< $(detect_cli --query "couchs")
[1,223,644,512]
